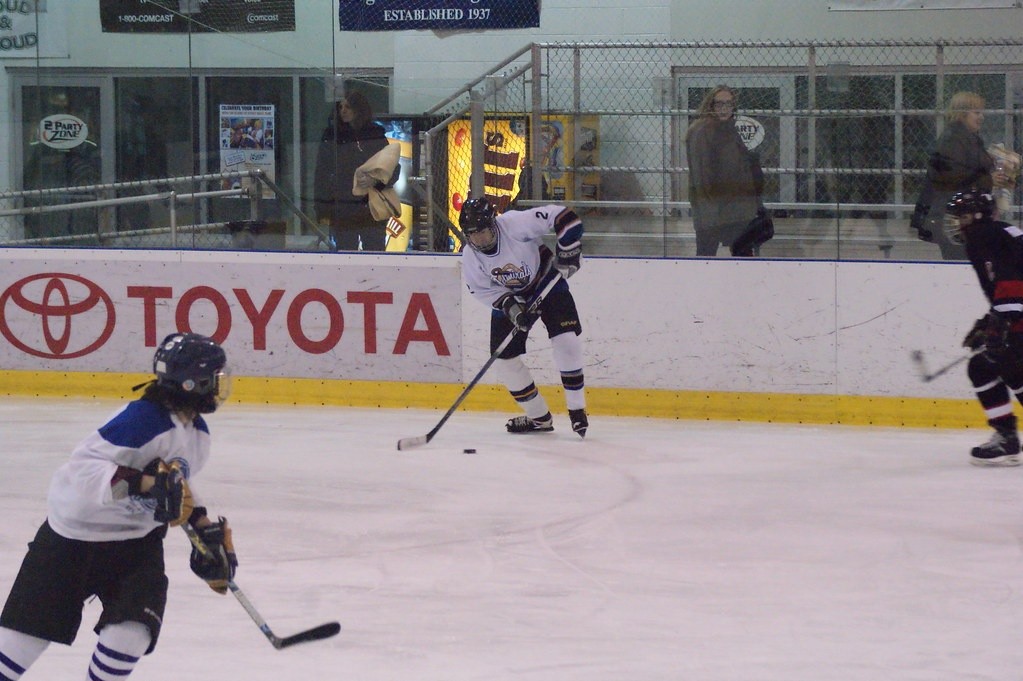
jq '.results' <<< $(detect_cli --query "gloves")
[963,314,992,352]
[555,241,582,279]
[984,310,1012,363]
[500,294,535,333]
[125,457,194,528]
[189,516,237,594]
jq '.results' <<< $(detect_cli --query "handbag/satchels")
[743,211,775,249]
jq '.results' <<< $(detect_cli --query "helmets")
[942,189,995,246]
[153,333,226,414]
[458,197,499,256]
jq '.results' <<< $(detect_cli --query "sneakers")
[970,415,1022,467]
[506,411,555,433]
[567,408,589,439]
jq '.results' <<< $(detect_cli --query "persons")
[459,200,589,440]
[223,140,228,147]
[313,93,400,251]
[684,85,775,258]
[267,121,272,128]
[0,333,237,681]
[940,192,1023,468]
[911,91,1004,262]
[64,90,186,233]
[265,131,271,138]
[229,119,261,151]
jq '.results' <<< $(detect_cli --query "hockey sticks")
[910,342,987,384]
[395,270,563,452]
[180,519,342,652]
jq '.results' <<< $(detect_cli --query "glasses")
[713,100,734,110]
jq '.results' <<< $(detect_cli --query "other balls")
[463,448,476,454]
